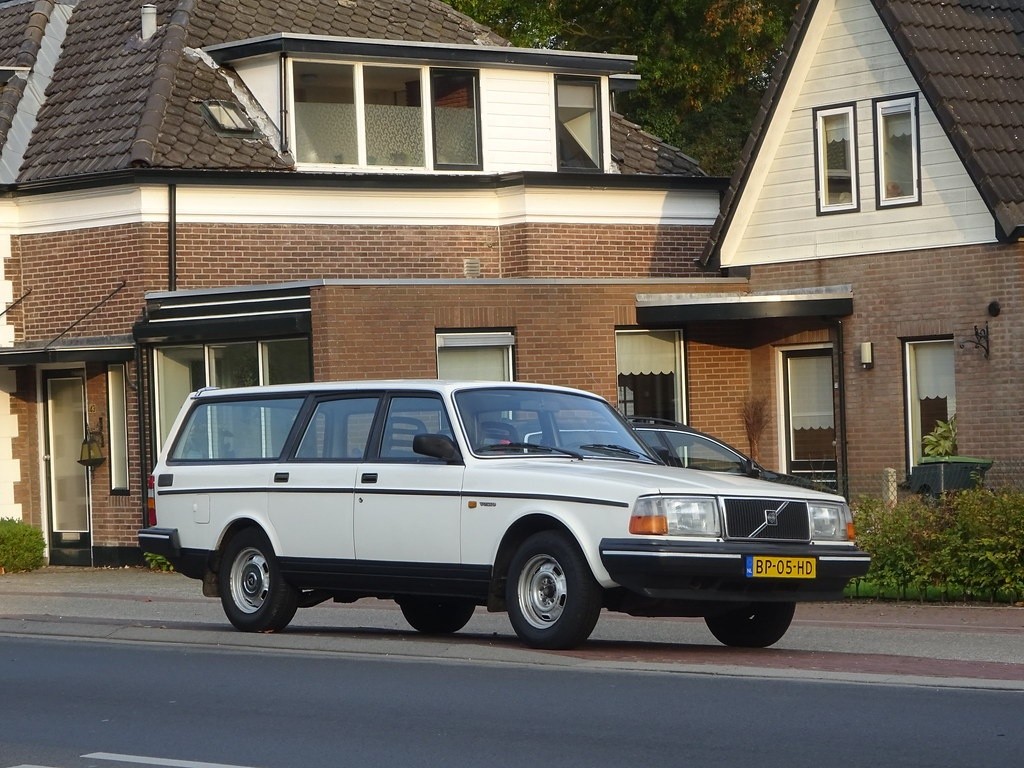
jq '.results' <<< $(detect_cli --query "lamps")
[76,415,104,472]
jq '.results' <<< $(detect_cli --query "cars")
[137,378,872,651]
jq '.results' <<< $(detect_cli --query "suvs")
[436,416,836,495]
